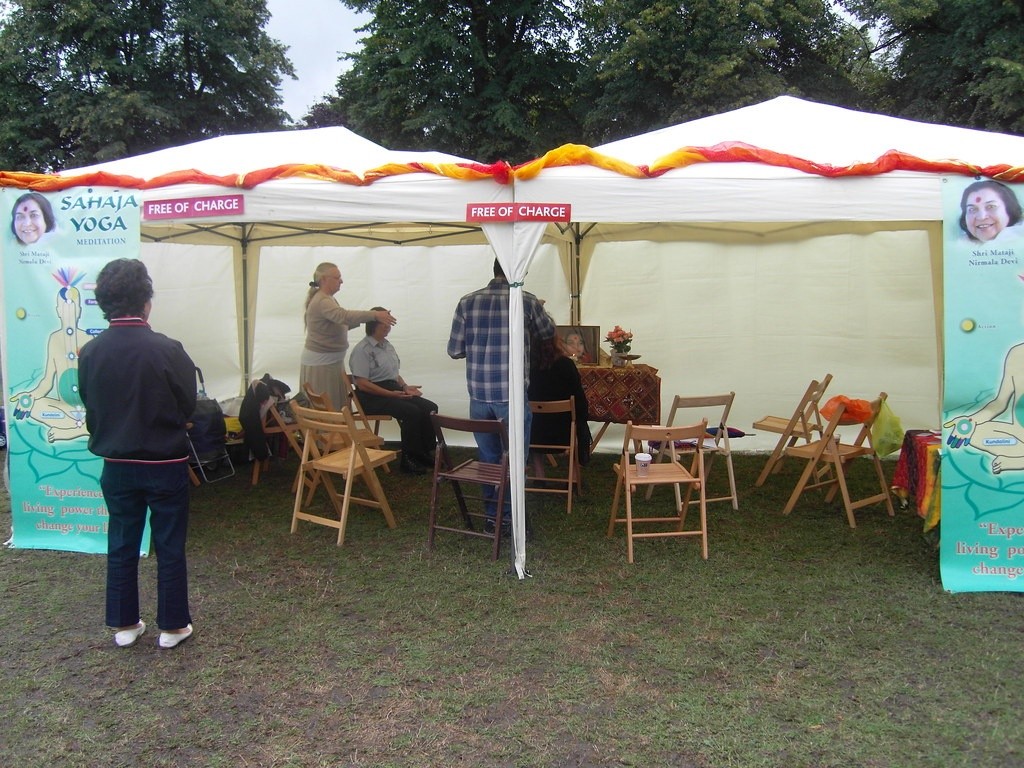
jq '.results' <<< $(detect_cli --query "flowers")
[603,326,633,354]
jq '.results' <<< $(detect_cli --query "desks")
[891,430,941,549]
[576,364,661,454]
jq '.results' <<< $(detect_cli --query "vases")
[611,349,627,367]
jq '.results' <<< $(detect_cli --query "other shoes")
[159,623,193,649]
[574,488,592,500]
[115,620,146,647]
[483,517,512,538]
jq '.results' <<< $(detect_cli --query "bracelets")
[401,384,407,388]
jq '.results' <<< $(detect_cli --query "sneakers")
[420,452,444,469]
[400,455,426,475]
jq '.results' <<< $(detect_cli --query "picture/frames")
[556,326,600,365]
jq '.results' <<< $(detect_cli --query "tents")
[0,95,1024,581]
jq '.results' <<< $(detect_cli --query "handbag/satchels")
[871,396,904,459]
[271,392,309,426]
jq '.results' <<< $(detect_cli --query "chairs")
[342,372,393,473]
[523,395,582,513]
[249,373,322,485]
[648,391,739,516]
[302,382,384,484]
[429,411,510,561]
[607,417,712,564]
[753,373,834,492]
[782,392,895,528]
[288,399,398,545]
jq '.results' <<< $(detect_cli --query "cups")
[634,452,652,477]
[825,434,840,450]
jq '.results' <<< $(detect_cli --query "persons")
[528,328,592,503]
[349,307,443,473]
[77,260,195,647]
[447,257,555,541]
[300,262,397,451]
[958,181,1024,242]
[11,192,55,245]
[558,331,591,362]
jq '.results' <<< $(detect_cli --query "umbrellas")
[704,427,757,438]
